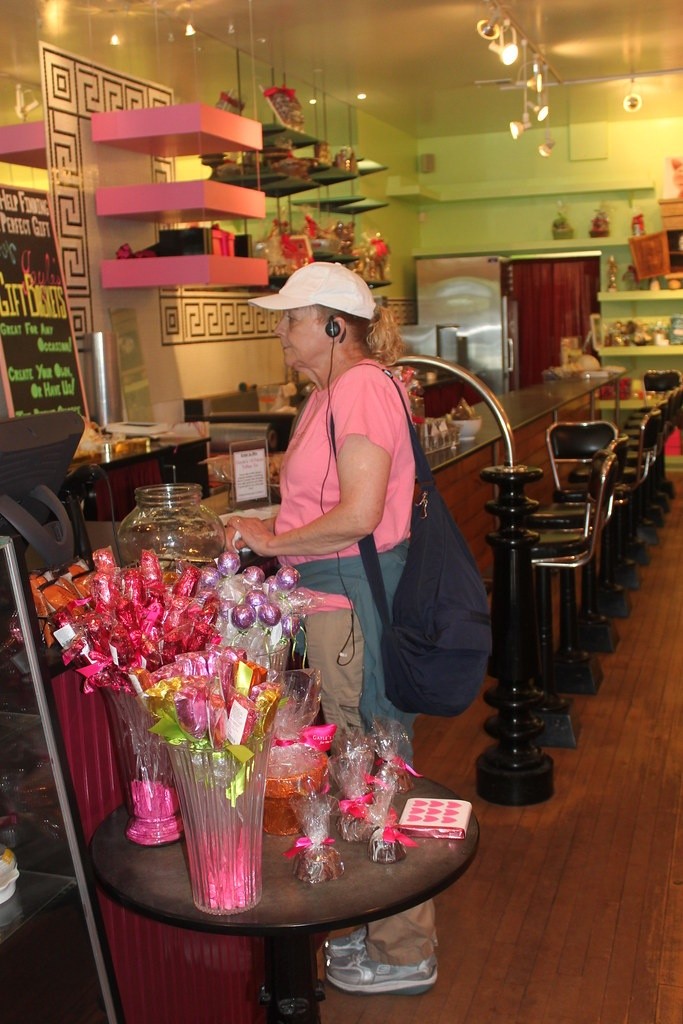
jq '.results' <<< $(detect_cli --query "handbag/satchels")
[330,365,491,717]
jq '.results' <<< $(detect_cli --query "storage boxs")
[160,228,254,259]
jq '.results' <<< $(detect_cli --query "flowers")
[142,645,285,751]
[43,548,221,697]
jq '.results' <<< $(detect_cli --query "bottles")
[117,483,227,569]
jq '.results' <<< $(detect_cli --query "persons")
[227,261,440,993]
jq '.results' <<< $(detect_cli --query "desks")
[88,752,479,1024]
[69,420,212,525]
[0,708,94,955]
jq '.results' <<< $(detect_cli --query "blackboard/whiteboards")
[0,184,91,424]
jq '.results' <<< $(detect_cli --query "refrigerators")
[415,255,518,396]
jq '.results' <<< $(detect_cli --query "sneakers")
[322,925,437,960]
[324,949,438,996]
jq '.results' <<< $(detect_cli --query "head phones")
[326,322,339,336]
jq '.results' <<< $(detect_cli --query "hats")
[248,262,376,320]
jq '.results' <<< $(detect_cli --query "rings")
[237,519,240,522]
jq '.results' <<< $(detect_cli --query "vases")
[163,722,271,915]
[108,689,185,847]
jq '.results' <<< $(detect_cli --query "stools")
[523,369,683,751]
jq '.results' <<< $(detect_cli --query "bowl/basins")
[263,752,328,835]
[447,420,481,441]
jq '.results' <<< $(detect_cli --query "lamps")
[473,10,642,157]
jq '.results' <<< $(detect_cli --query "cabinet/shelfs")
[92,101,268,288]
[596,287,683,472]
[386,177,655,258]
[418,368,487,420]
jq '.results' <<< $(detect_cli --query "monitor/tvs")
[0,411,85,542]
